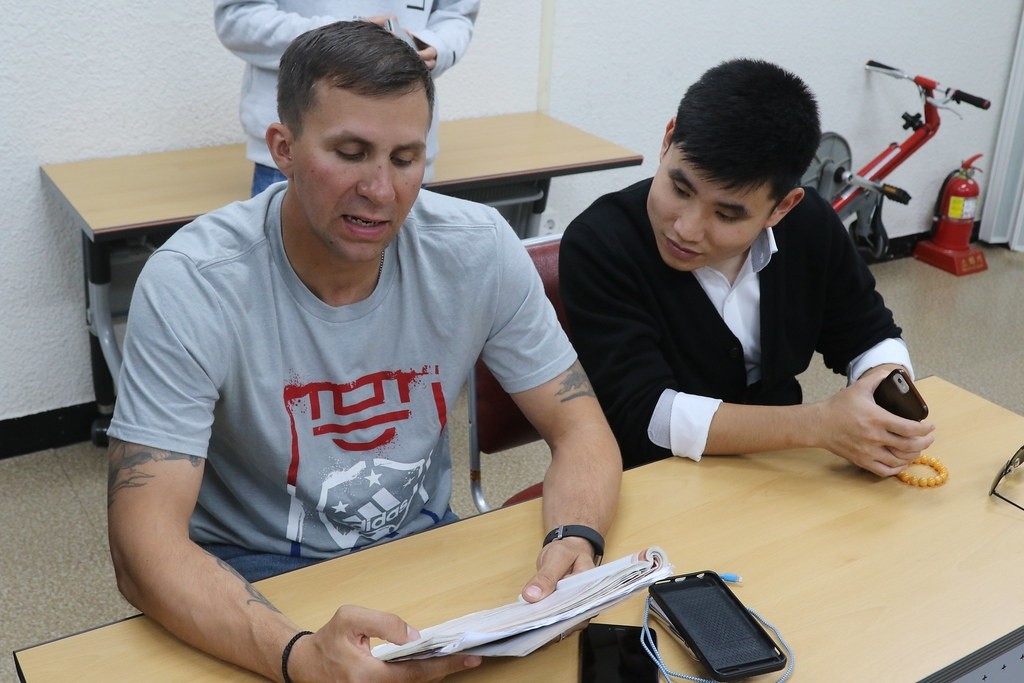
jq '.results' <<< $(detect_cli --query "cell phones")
[579,623,658,683]
[872,369,928,423]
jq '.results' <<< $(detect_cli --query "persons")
[215,0,480,202]
[110,19,624,683]
[560,58,934,478]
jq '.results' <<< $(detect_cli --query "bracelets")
[282,630,314,683]
[542,524,605,566]
[895,454,947,487]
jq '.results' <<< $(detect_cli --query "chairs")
[464,234,562,512]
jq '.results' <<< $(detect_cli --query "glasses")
[989,445,1024,511]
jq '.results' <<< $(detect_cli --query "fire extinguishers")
[933,154,986,248]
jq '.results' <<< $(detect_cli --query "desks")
[12,372,1024,683]
[45,110,644,452]
